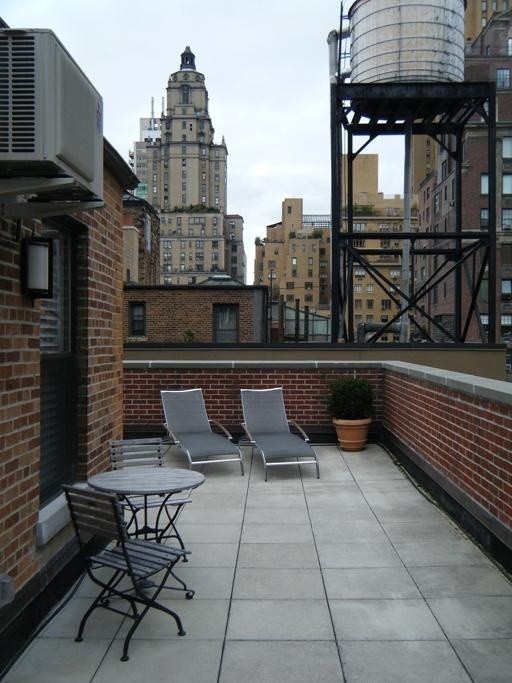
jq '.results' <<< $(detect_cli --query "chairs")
[62,484,192,661]
[160,388,244,476]
[238,387,320,481]
[109,437,192,562]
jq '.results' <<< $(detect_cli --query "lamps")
[21,237,54,298]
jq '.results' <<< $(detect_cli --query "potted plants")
[325,374,377,451]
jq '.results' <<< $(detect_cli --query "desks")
[87,466,205,607]
